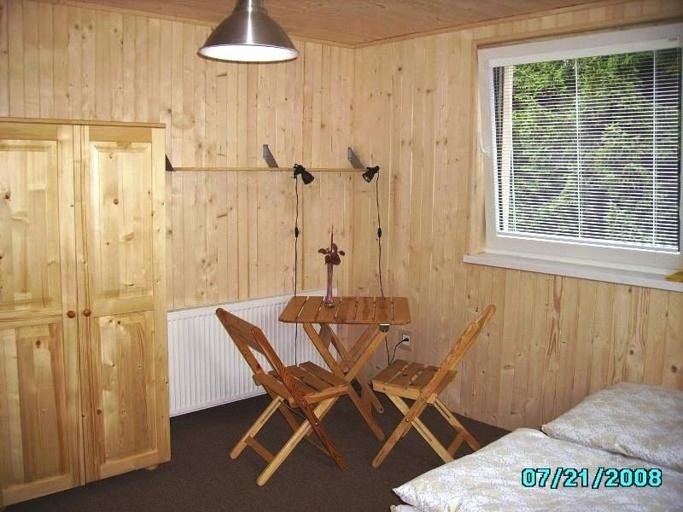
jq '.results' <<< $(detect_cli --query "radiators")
[168,289,338,416]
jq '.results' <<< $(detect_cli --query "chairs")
[370,303,494,469]
[215,307,351,487]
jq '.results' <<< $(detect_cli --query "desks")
[278,295,412,439]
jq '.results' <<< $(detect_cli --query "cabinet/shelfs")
[0,116,173,508]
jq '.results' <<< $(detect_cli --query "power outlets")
[401,334,411,347]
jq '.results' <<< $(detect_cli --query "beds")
[542,379,683,473]
[389,428,682,512]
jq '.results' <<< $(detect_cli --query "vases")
[322,264,333,308]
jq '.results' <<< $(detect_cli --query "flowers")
[317,243,345,265]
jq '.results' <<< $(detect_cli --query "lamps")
[362,165,409,367]
[290,163,315,362]
[196,0,300,64]
